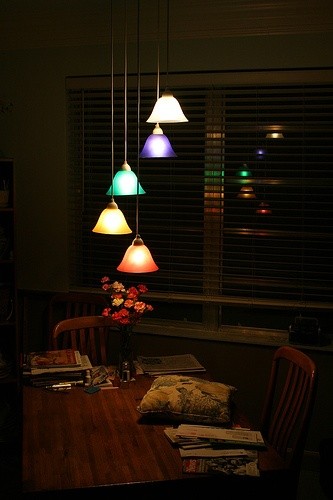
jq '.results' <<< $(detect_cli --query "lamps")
[145,0,189,124]
[91,0,133,234]
[105,0,147,196]
[116,0,160,272]
[139,0,180,159]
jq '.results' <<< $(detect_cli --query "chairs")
[258,343,318,500]
[50,316,119,368]
[48,292,110,357]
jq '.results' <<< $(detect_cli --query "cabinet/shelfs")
[0,157,22,500]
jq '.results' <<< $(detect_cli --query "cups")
[0,190,9,208]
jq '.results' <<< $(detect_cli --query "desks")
[21,363,287,500]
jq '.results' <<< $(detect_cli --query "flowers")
[98,275,157,337]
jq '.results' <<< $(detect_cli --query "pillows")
[136,373,238,425]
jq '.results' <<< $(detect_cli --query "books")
[163,424,265,478]
[135,353,205,374]
[28,349,93,388]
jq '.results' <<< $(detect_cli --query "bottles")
[84,370,91,386]
[59,380,83,386]
[122,360,130,383]
[45,383,72,393]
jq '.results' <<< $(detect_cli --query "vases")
[115,324,134,369]
